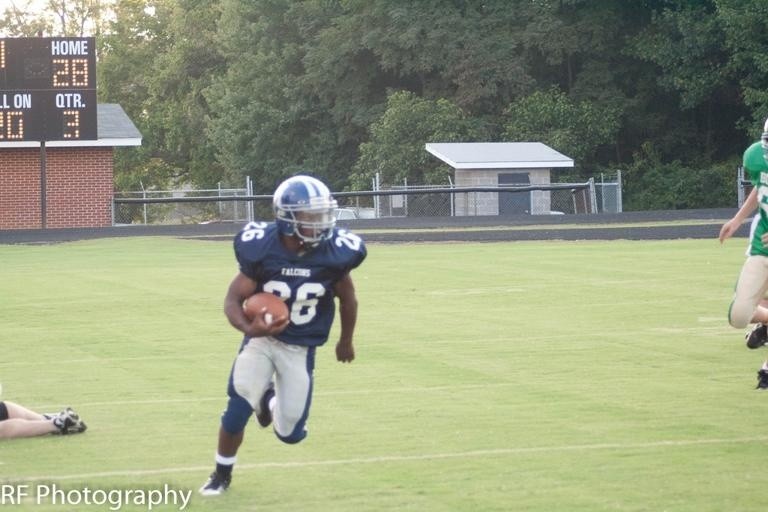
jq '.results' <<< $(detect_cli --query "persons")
[718,117,768,391]
[199,174,368,497]
[0,401,86,439]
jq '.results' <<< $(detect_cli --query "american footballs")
[242,292,288,323]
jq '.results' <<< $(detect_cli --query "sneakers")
[53,407,87,434]
[198,472,231,496]
[746,326,767,348]
[256,381,274,428]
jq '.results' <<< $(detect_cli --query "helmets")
[272,175,340,248]
